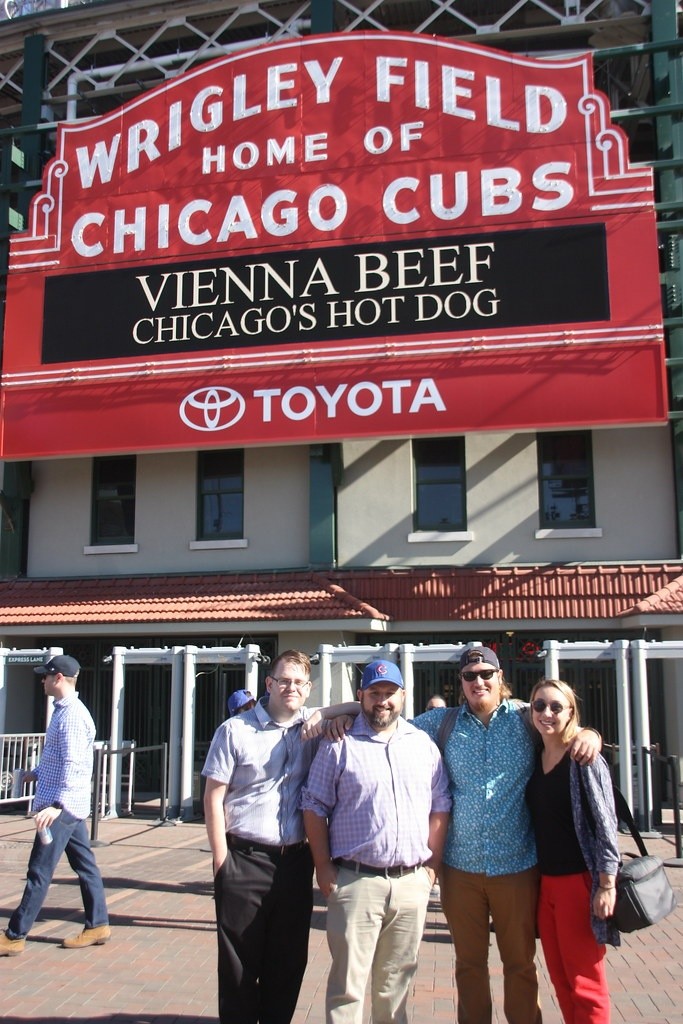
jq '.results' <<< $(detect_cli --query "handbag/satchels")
[608,856,677,933]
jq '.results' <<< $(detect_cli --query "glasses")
[268,673,308,690]
[459,668,501,682]
[43,672,67,680]
[530,700,570,714]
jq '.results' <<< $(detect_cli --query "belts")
[225,833,309,856]
[333,856,422,879]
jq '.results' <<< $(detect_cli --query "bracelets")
[599,885,615,890]
[583,727,600,738]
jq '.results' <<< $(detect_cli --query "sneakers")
[62,925,111,947]
[0,928,25,956]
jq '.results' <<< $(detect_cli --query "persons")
[200,645,620,1024]
[0,655,111,957]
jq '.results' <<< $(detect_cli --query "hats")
[227,690,254,716]
[458,646,500,669]
[34,655,81,677]
[360,660,404,692]
